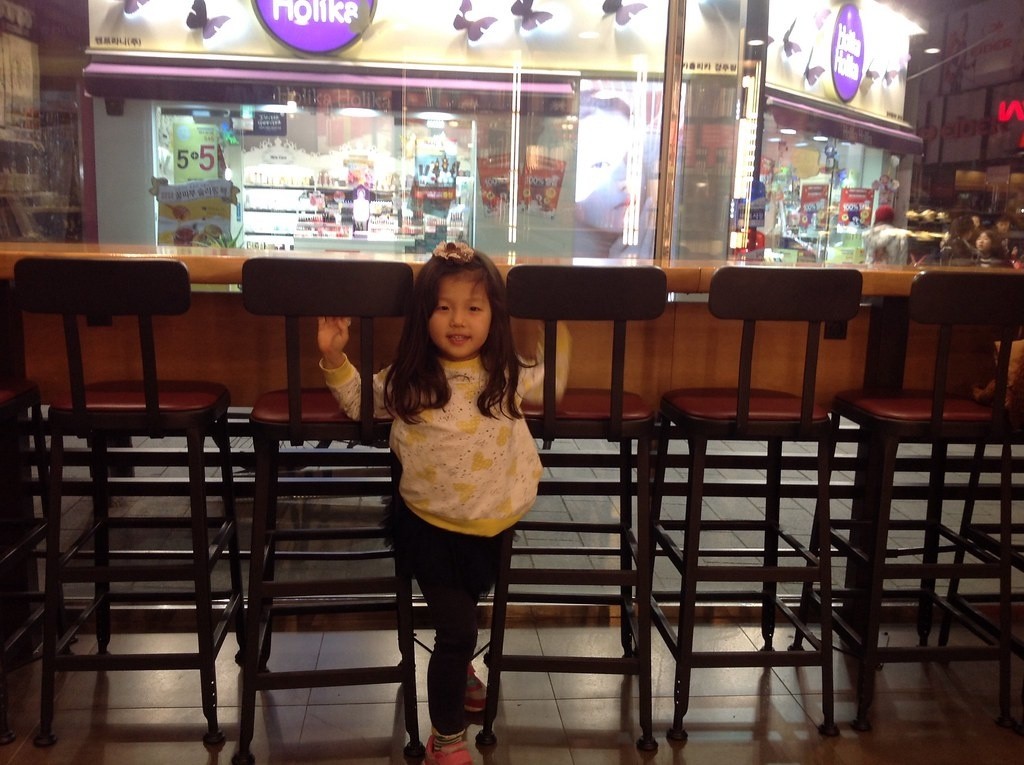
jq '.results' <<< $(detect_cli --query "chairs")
[634,266,865,738]
[789,270,1024,732]
[475,265,666,752]
[235,258,415,759]
[14,254,244,755]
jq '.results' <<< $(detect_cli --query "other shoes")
[425,736,474,764]
[462,663,488,712]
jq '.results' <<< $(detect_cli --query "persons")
[872,175,895,207]
[939,213,1018,269]
[860,205,908,266]
[572,89,648,258]
[318,241,571,765]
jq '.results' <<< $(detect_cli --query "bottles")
[418,157,460,187]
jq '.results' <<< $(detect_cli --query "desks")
[0,241,1024,424]
